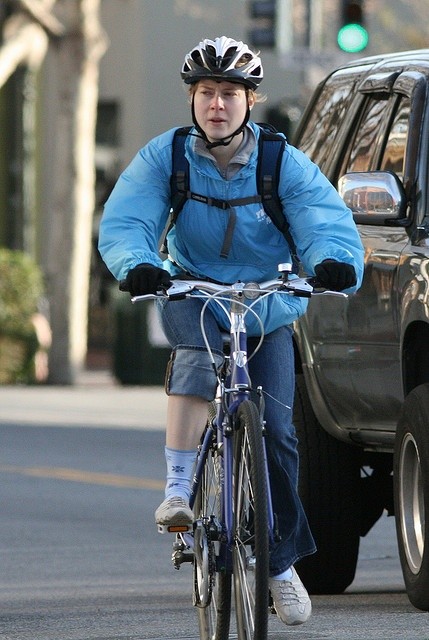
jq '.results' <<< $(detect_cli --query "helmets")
[179,38,263,91]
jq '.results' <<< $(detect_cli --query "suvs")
[287,49,429,614]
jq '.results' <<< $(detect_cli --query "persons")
[98,36,365,627]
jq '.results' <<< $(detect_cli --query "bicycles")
[118,262,349,640]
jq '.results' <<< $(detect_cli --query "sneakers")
[155,493,195,528]
[268,562,312,627]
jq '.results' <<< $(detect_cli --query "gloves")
[124,262,173,299]
[307,258,358,292]
